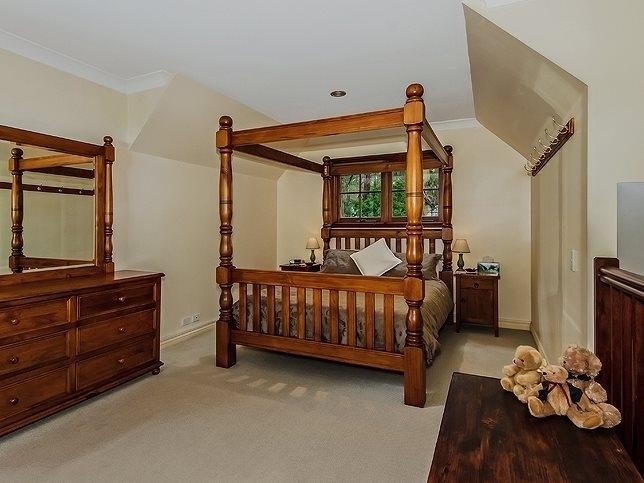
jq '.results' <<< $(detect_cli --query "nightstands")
[279,262,321,273]
[453,271,500,337]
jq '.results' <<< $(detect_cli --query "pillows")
[349,238,402,277]
[321,248,442,280]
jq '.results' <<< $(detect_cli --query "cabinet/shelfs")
[0,270,165,439]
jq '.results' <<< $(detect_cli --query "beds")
[8,148,96,273]
[215,83,454,408]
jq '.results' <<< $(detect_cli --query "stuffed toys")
[500,344,543,397]
[517,364,570,417]
[559,344,622,430]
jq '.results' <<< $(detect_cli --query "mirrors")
[0,125,106,285]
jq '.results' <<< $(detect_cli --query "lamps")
[305,237,320,264]
[452,239,470,271]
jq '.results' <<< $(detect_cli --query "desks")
[426,373,644,483]
